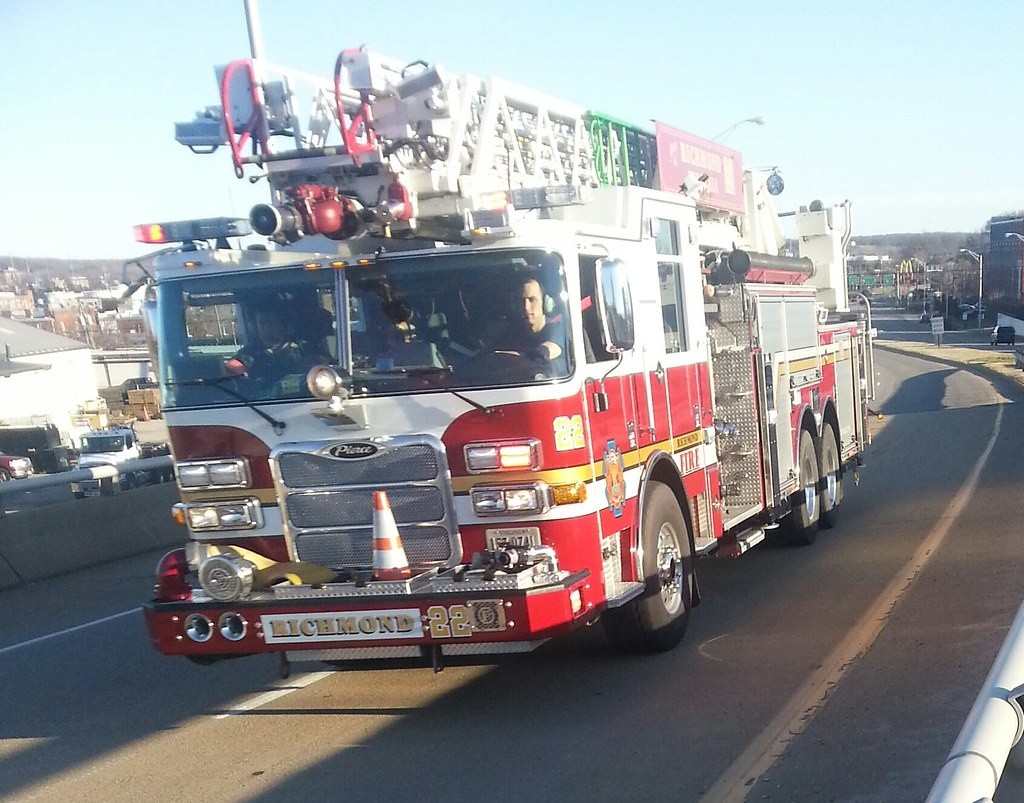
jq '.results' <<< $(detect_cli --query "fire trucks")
[144,24,878,674]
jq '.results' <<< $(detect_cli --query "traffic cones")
[357,491,419,582]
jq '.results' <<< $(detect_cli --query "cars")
[958,302,986,322]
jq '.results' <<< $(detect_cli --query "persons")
[234,305,312,385]
[487,268,595,378]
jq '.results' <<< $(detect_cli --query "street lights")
[911,257,926,316]
[960,248,983,328]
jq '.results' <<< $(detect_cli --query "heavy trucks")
[71,427,174,501]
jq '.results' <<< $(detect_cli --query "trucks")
[0,452,34,486]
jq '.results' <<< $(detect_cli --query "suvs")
[991,324,1015,346]
